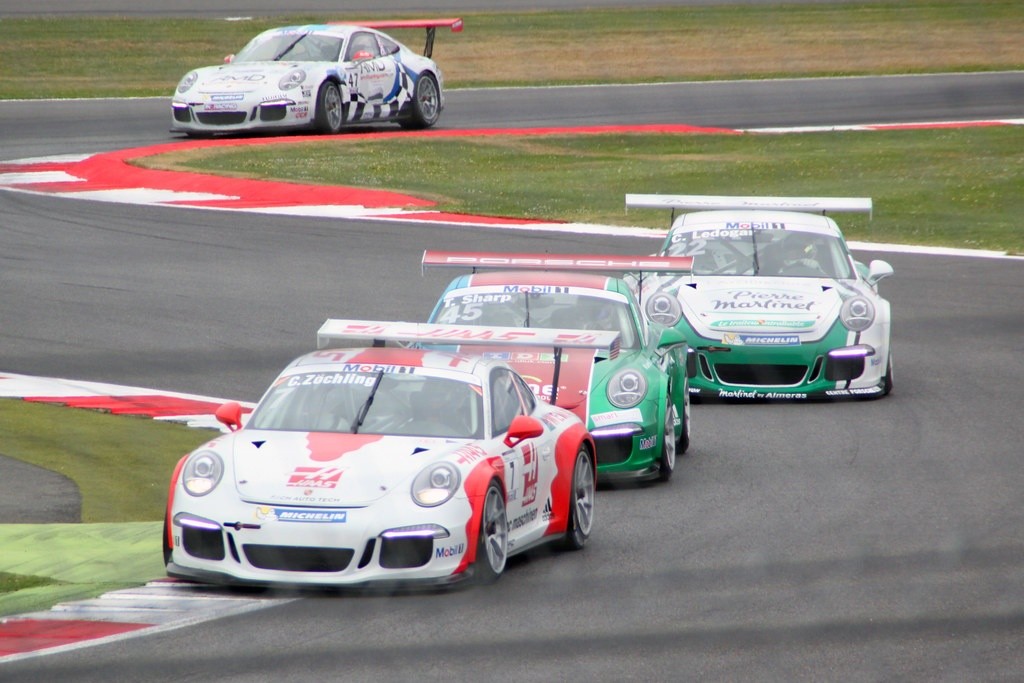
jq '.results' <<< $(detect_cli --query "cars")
[619,189,896,403]
[159,317,623,591]
[408,249,696,487]
[167,16,465,139]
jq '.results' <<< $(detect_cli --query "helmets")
[577,295,616,331]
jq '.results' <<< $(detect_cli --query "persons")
[576,297,614,329]
[412,381,471,435]
[761,233,831,275]
